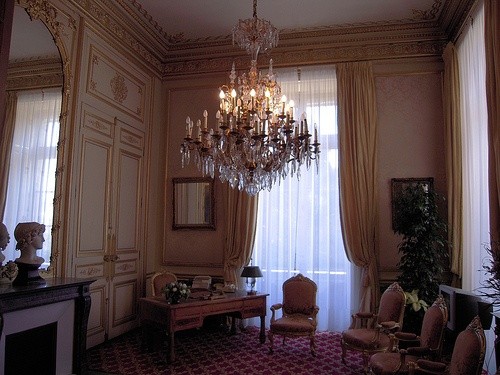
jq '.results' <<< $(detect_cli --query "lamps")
[179,0,321,199]
[239,261,263,296]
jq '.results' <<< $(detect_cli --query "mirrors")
[390,176,434,230]
[172,176,216,232]
[1,4,72,281]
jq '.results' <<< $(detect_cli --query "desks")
[137,288,270,362]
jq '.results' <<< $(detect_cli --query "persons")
[0,222,11,251]
[14,221,46,265]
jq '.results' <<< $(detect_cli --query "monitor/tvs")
[439,285,493,330]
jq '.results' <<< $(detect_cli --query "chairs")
[268,273,319,355]
[341,282,487,375]
[151,269,177,296]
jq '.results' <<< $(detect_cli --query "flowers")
[163,280,193,304]
